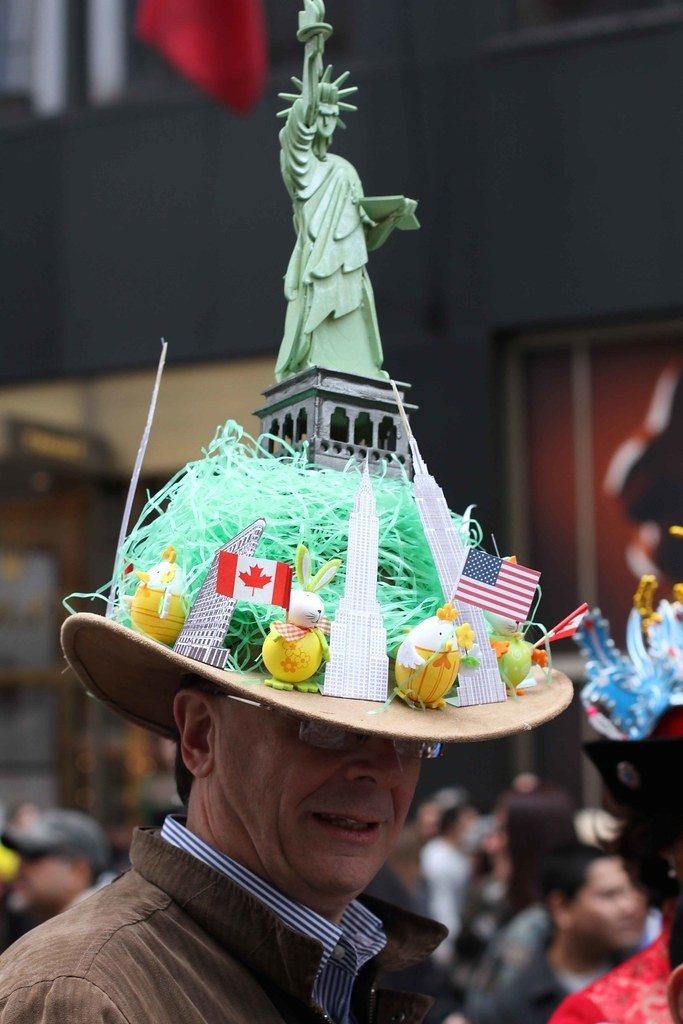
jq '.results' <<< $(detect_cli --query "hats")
[578,523,683,809]
[57,0,573,744]
[1,808,112,875]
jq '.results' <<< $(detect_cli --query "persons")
[276,0,420,379]
[369,731,683,1024]
[0,657,451,1024]
[0,799,139,954]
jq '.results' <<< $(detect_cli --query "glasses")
[205,689,448,760]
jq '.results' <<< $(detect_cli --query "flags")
[539,602,588,647]
[216,550,294,612]
[450,547,541,624]
